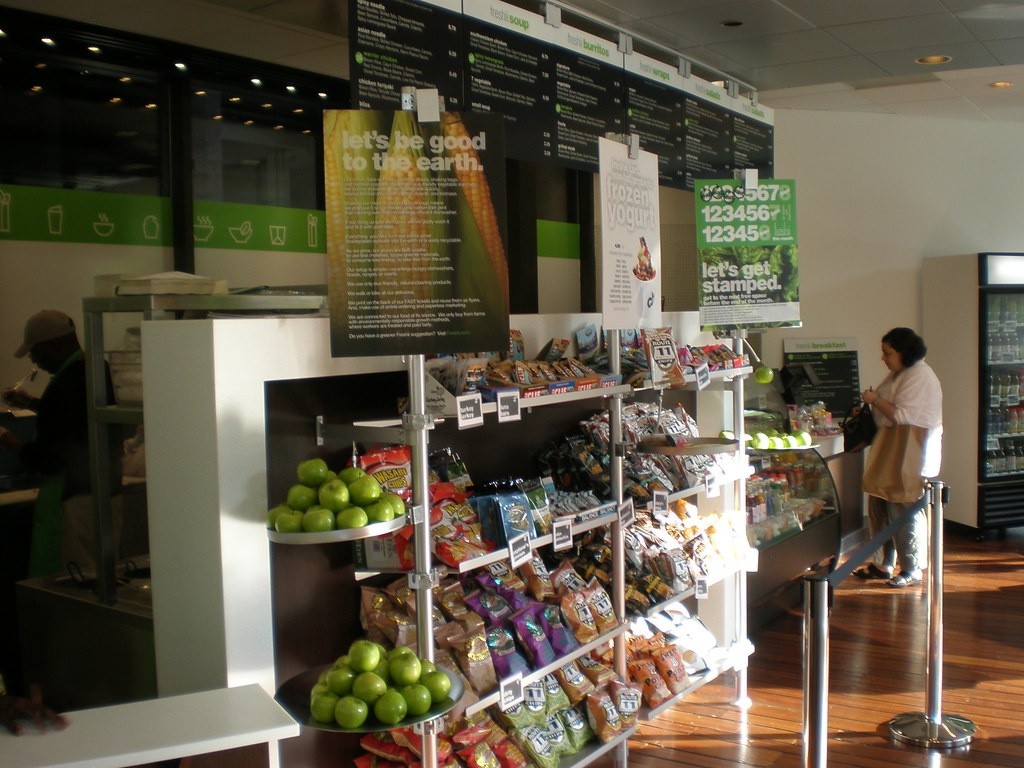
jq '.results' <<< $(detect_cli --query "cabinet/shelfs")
[351,329,759,768]
[85,288,328,612]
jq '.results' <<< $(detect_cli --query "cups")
[633,266,656,320]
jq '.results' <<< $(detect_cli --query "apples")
[754,364,773,384]
[718,429,812,449]
[268,458,405,533]
[309,640,450,729]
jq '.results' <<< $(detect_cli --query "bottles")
[989,371,1024,402]
[745,473,790,524]
[986,408,1024,435]
[988,329,1020,362]
[985,441,1024,473]
[790,463,829,499]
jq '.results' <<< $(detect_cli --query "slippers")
[852,563,893,579]
[888,571,923,588]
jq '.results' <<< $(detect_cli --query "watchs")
[872,397,881,407]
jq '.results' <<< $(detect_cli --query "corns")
[322,108,509,339]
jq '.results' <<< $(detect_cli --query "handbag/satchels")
[842,403,878,454]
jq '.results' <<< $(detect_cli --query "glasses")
[27,352,37,358]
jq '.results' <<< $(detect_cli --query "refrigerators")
[922,252,1024,529]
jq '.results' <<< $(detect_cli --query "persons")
[850,326,945,587]
[0,310,121,581]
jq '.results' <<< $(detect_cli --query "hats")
[14,310,77,359]
